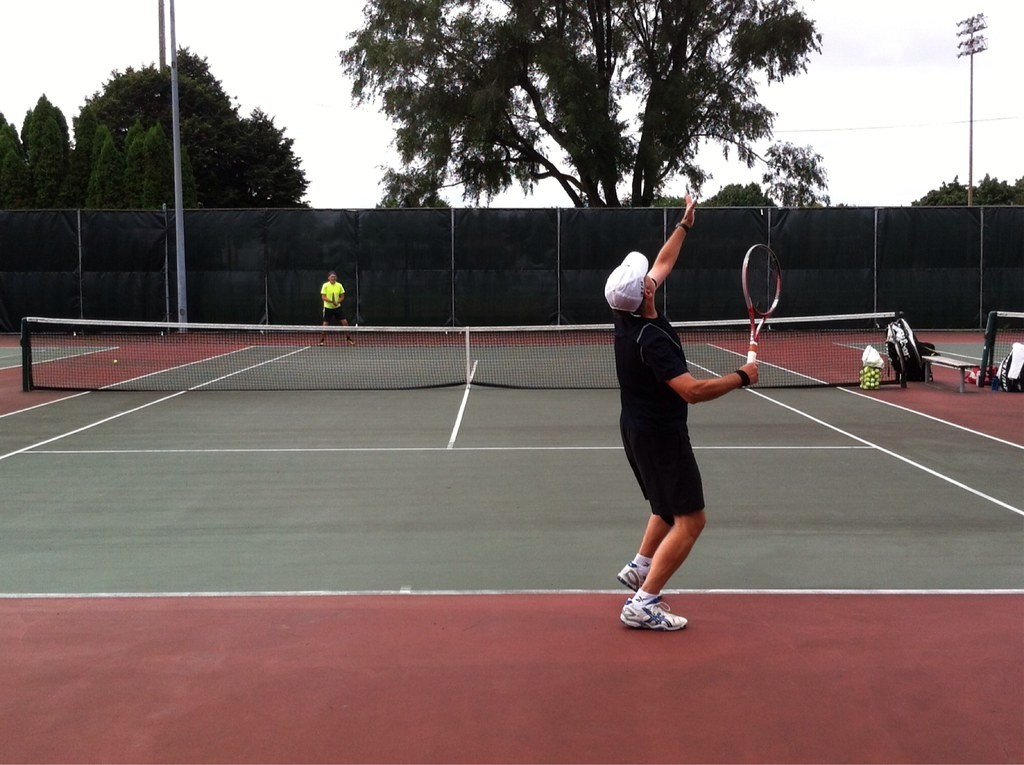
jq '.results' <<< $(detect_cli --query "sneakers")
[616,560,649,591]
[620,595,688,631]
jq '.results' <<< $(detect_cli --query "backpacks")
[885,318,936,382]
[992,342,1024,392]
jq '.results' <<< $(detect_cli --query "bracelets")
[676,223,690,234]
[735,370,750,388]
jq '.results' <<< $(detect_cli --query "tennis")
[860,364,883,389]
[114,359,118,364]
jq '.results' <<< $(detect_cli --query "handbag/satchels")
[861,345,885,369]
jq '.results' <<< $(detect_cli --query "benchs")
[922,356,980,392]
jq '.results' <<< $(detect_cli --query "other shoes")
[320,339,324,345]
[347,338,355,345]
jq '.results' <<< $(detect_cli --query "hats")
[605,251,649,312]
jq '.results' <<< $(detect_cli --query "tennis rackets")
[332,292,337,307]
[741,243,780,377]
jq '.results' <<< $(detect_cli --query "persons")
[605,193,759,632]
[317,270,355,347]
